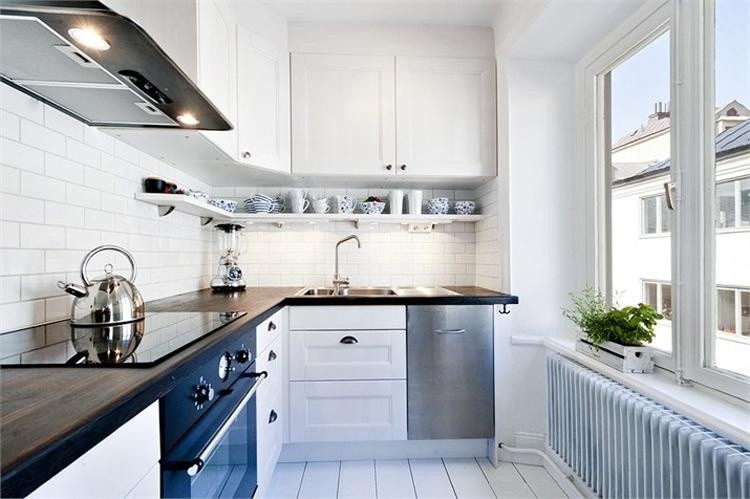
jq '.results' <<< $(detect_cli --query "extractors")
[0,0,235,132]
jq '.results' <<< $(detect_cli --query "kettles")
[56,244,145,327]
[64,319,145,364]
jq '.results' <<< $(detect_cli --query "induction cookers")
[0,312,256,457]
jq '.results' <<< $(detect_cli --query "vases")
[575,330,655,374]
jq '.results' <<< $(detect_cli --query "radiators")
[544,350,750,499]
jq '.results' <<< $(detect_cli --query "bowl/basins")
[190,192,209,204]
[208,199,241,213]
[423,197,450,214]
[453,199,476,214]
[358,202,386,214]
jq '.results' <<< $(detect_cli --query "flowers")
[561,283,664,355]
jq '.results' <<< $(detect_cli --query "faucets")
[330,234,361,288]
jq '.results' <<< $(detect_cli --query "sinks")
[295,287,400,297]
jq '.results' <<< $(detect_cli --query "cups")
[292,198,310,214]
[243,191,287,214]
[312,197,331,214]
[335,193,358,214]
[388,190,404,214]
[408,190,422,214]
[288,189,307,201]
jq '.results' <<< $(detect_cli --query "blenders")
[209,220,247,290]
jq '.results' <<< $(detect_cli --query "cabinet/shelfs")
[286,296,408,444]
[289,52,396,189]
[98,0,236,162]
[256,297,289,497]
[405,297,495,441]
[394,55,497,192]
[0,399,160,499]
[159,318,258,499]
[173,24,292,191]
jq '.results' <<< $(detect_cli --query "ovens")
[158,359,268,498]
